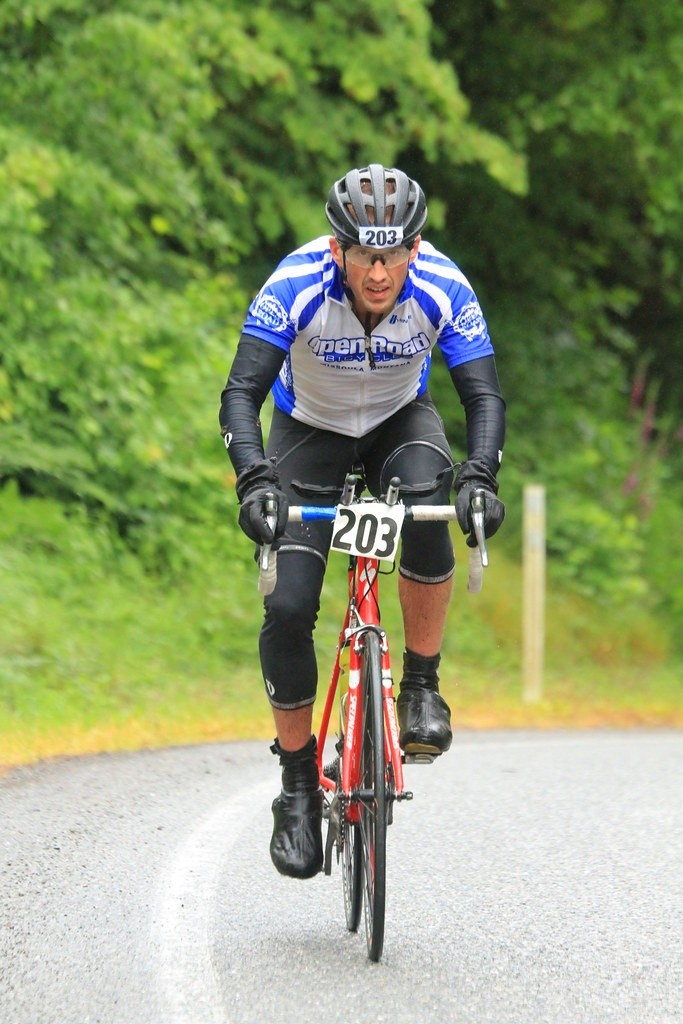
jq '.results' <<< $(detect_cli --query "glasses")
[336,239,416,269]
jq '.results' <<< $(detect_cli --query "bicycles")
[256,463,489,962]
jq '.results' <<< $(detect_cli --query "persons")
[219,165,507,879]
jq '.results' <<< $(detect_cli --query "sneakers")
[271,784,324,879]
[397,689,452,754]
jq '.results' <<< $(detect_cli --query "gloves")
[456,479,505,547]
[239,482,288,551]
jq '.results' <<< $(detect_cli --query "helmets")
[324,164,428,248]
